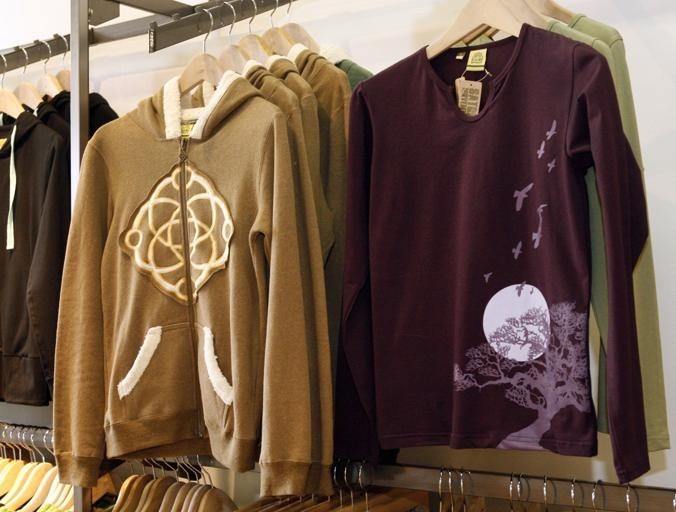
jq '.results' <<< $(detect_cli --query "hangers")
[425,0,573,63]
[0,34,75,120]
[178,0,324,99]
